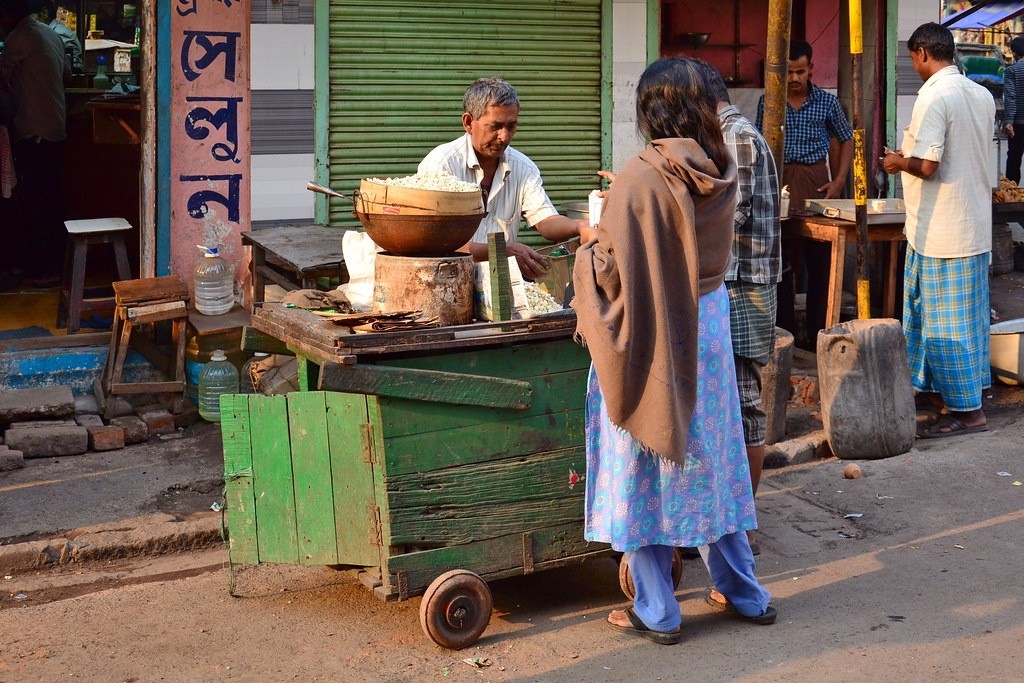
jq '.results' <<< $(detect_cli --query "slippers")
[605,606,681,644]
[917,415,990,438]
[707,594,777,625]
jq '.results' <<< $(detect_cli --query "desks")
[784,216,906,362]
[241,225,360,303]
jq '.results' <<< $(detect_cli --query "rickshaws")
[954,42,1005,122]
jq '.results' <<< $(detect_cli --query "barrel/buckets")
[817,318,918,457]
[988,222,1014,275]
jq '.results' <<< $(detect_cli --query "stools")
[57,217,133,335]
[94,274,189,419]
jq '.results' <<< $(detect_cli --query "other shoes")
[750,545,760,556]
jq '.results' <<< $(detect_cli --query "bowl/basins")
[562,200,589,219]
[89,30,105,40]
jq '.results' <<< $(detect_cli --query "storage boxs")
[535,236,580,307]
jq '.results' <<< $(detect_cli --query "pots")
[371,251,476,325]
[989,318,1024,385]
[353,188,489,255]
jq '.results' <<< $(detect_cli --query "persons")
[568,56,777,645]
[418,76,589,280]
[880,21,997,439]
[0,0,85,289]
[1003,36,1024,186]
[755,39,854,343]
[688,59,784,556]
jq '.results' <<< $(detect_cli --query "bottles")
[198,350,239,421]
[241,351,269,395]
[94,56,105,89]
[193,243,235,315]
[780,185,792,218]
